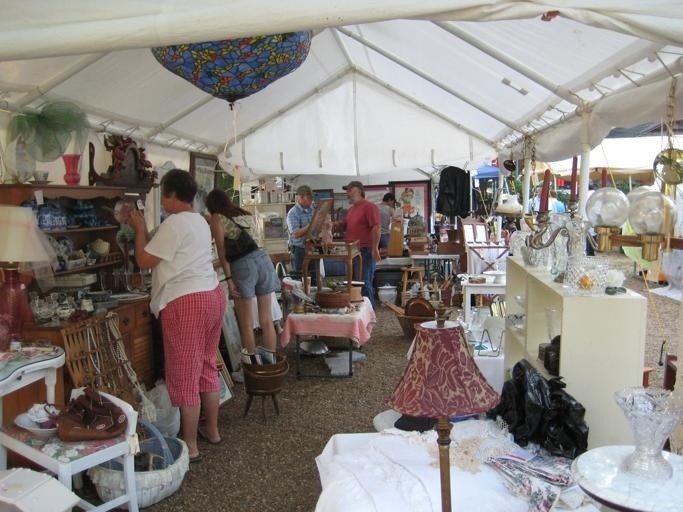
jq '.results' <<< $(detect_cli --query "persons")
[286,186,316,287]
[550,190,565,212]
[529,186,553,212]
[576,181,597,256]
[375,193,399,249]
[126,169,226,460]
[204,189,277,382]
[331,181,382,309]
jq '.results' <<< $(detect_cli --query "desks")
[462,274,507,325]
[280,296,376,380]
[303,239,362,302]
[376,254,460,306]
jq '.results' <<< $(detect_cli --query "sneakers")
[230,367,246,383]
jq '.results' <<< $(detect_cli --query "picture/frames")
[219,371,235,408]
[312,179,431,236]
[153,151,217,226]
[463,223,486,243]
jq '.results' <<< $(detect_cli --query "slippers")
[198,423,226,446]
[188,452,203,463]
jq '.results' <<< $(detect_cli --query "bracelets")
[225,276,232,281]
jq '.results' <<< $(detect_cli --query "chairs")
[0,344,139,512]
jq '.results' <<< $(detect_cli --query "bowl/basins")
[13,412,59,438]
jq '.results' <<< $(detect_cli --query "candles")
[539,156,607,213]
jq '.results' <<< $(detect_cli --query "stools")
[400,267,425,308]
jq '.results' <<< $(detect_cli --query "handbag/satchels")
[224,230,258,263]
[43,387,129,442]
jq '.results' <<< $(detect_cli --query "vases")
[63,154,80,185]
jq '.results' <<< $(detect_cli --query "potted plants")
[315,281,350,307]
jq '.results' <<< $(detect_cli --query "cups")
[613,385,682,483]
[34,169,49,181]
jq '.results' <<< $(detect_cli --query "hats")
[295,185,311,195]
[342,181,364,190]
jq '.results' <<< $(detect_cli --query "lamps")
[383,303,502,512]
[0,205,56,344]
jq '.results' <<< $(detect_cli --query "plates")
[571,444,682,512]
[110,292,150,302]
[28,181,54,184]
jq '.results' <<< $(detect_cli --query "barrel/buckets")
[242,354,290,391]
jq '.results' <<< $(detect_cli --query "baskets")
[85,417,190,509]
[397,315,448,339]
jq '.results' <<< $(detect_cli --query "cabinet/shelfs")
[0,184,152,424]
[503,257,648,451]
